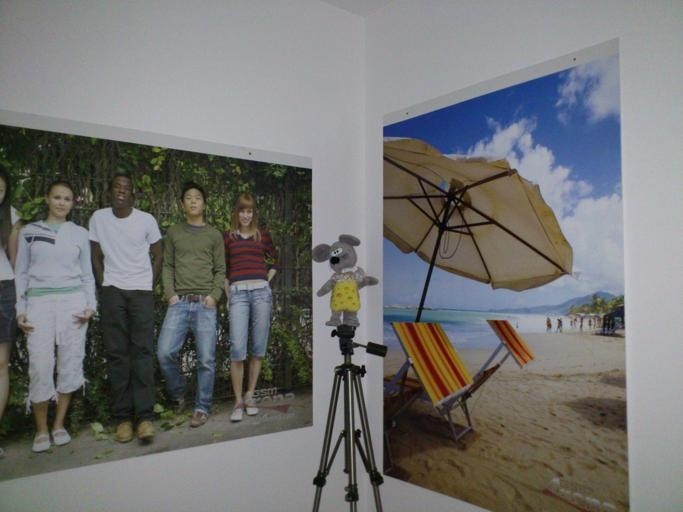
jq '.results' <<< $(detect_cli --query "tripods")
[313,326,388,512]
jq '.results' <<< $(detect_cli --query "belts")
[179,294,205,302]
[230,280,269,292]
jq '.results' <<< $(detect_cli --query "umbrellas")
[384,136,573,394]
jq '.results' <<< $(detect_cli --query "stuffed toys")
[311,234,378,326]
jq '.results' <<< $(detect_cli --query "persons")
[0,172,22,452]
[14,180,97,452]
[545,316,602,335]
[157,185,225,427]
[88,174,163,442]
[222,194,278,421]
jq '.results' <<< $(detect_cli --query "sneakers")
[52,426,71,447]
[116,420,133,443]
[32,429,50,453]
[137,419,155,440]
[244,392,259,415]
[229,401,248,423]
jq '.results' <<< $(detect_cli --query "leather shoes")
[173,391,187,415]
[190,407,209,427]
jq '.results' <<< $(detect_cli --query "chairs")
[384,320,535,423]
[391,315,502,445]
[593,323,621,336]
